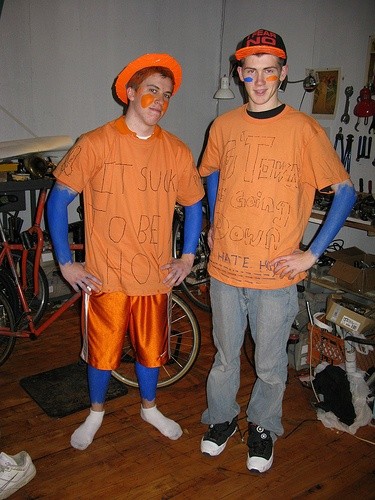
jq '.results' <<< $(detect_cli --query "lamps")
[213,74,235,99]
[277,73,317,93]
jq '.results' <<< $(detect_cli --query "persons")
[198,27,357,472]
[43,50,204,451]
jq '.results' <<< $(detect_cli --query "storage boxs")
[308,316,375,372]
[325,247,375,294]
[326,295,375,334]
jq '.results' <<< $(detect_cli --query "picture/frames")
[309,66,341,118]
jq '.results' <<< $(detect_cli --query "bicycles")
[0,159,214,389]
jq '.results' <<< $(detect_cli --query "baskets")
[306,315,375,375]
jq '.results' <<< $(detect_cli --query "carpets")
[17,359,131,417]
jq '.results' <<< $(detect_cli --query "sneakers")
[0,450,37,500]
[241,421,277,473]
[200,415,242,456]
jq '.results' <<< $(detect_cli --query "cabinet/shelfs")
[298,209,375,303]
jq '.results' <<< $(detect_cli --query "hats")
[115,52,182,105]
[236,28,287,62]
